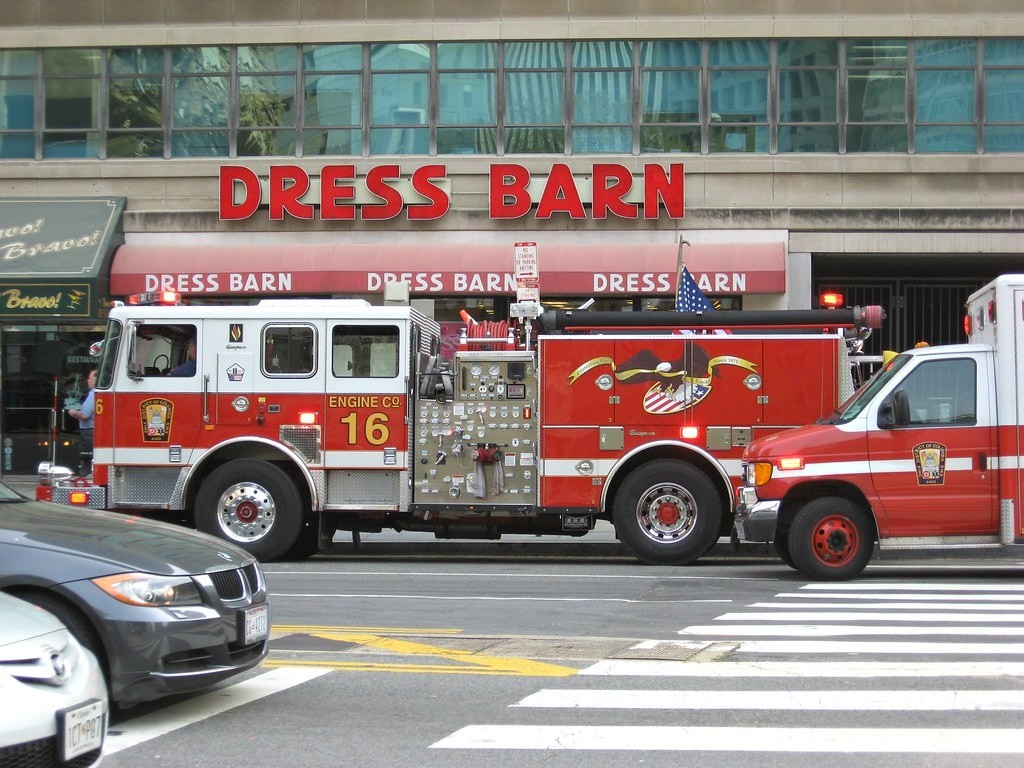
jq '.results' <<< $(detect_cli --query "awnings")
[109,244,787,295]
[0,196,127,322]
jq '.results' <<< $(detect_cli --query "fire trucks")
[37,290,903,568]
[731,273,1024,583]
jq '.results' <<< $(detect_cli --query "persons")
[68,370,98,480]
[168,337,197,377]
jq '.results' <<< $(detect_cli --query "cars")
[0,588,109,768]
[0,481,272,710]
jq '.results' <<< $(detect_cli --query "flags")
[675,264,732,335]
